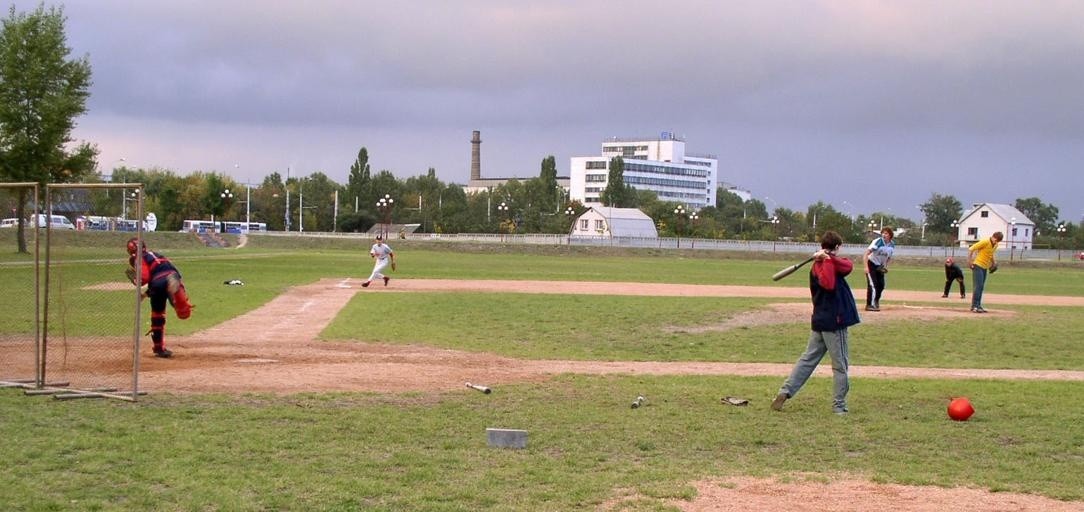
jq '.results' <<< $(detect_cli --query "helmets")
[126,237,146,257]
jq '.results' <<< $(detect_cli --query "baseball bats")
[631,395,644,409]
[771,255,816,282]
[465,382,492,395]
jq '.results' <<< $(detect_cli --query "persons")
[863,227,895,311]
[967,232,1003,313]
[941,257,965,299]
[769,230,860,414]
[361,235,396,287]
[126,237,192,358]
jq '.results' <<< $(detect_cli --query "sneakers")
[383,276,390,286]
[865,305,880,312]
[970,307,989,313]
[152,346,172,357]
[770,392,788,411]
[361,280,371,288]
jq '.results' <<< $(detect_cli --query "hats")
[945,257,954,264]
[376,235,382,240]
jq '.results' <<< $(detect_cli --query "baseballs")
[272,194,278,198]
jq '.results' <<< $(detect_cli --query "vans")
[1,217,30,228]
[30,214,75,229]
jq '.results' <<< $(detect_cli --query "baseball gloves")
[876,264,888,273]
[126,267,138,286]
[989,263,997,273]
[392,263,396,272]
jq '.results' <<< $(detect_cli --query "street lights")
[128,164,137,170]
[948,218,960,257]
[868,220,878,243]
[131,188,139,218]
[497,202,509,243]
[672,203,685,248]
[117,155,128,220]
[300,205,320,232]
[688,211,698,248]
[220,189,233,221]
[376,199,387,239]
[565,206,575,245]
[383,194,394,239]
[1054,223,1067,261]
[1006,215,1019,259]
[771,216,780,253]
[762,195,933,240]
[272,193,286,218]
[233,163,252,232]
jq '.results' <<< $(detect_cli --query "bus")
[77,213,147,230]
[181,219,267,232]
